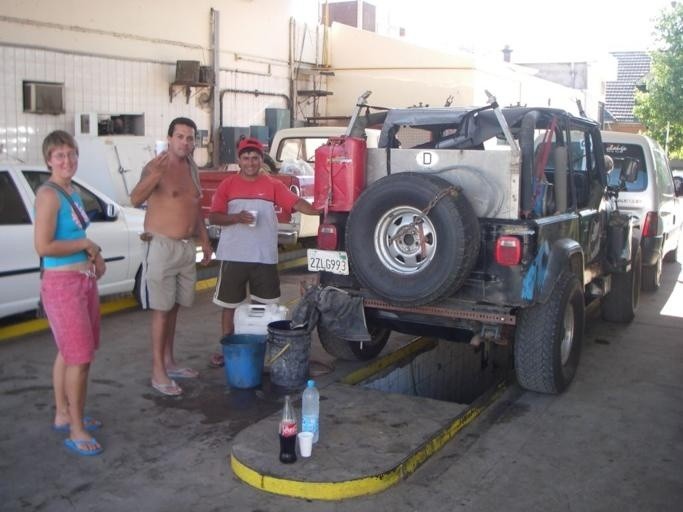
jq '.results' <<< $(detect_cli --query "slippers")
[53,418,101,431]
[208,352,223,368]
[65,437,104,455]
[167,366,198,377]
[153,380,182,396]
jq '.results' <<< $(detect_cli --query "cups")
[247,211,258,227]
[297,431,313,457]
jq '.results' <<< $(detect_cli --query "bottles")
[300,379,320,442]
[279,394,296,463]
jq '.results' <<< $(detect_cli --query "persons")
[128,118,213,398]
[32,128,108,455]
[207,137,330,370]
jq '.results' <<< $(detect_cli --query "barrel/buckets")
[312,136,366,212]
[220,334,267,389]
[266,320,312,389]
[233,303,289,368]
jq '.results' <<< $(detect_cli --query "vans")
[530,127,683,293]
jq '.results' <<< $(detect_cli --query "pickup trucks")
[188,124,381,246]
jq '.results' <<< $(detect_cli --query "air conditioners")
[23,83,64,115]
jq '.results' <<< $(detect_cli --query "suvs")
[319,107,641,395]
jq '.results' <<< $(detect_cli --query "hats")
[236,138,263,161]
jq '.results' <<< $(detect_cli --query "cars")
[0,166,149,317]
[666,157,683,195]
